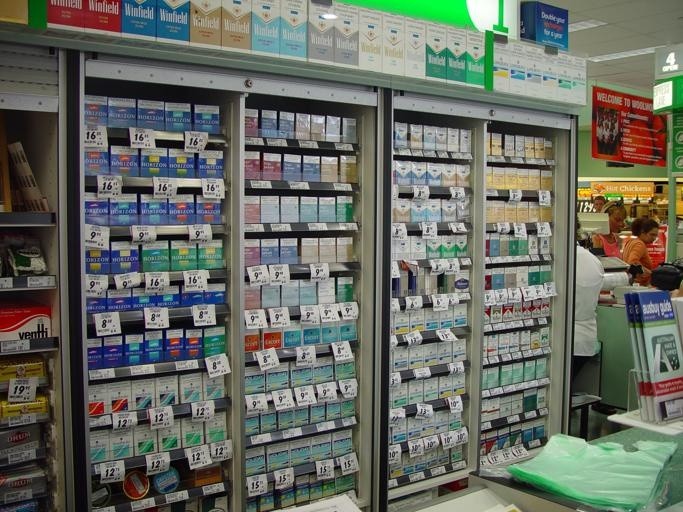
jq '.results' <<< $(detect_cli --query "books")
[623,291,683,424]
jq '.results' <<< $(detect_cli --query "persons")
[591,194,605,212]
[621,216,660,285]
[569,208,606,385]
[591,200,624,260]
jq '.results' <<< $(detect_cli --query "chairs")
[574,334,606,442]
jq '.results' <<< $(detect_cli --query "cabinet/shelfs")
[1,36,75,507]
[80,56,388,512]
[392,102,575,506]
